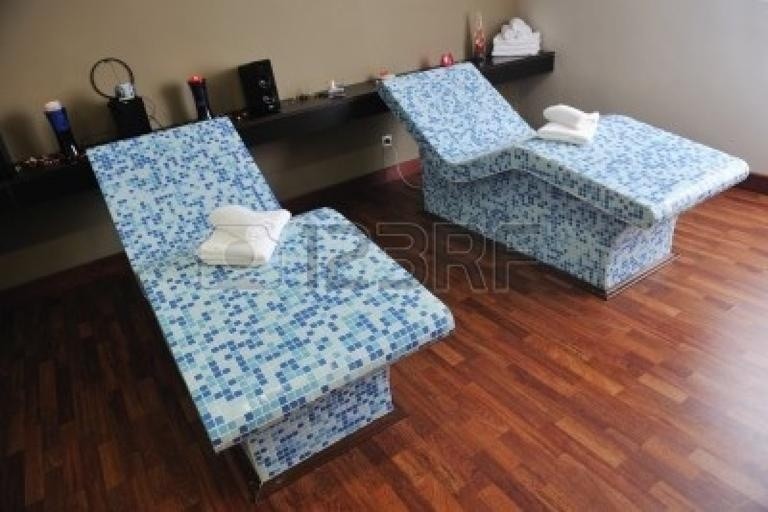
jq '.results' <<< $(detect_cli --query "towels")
[195,204,290,267]
[543,103,586,128]
[538,112,599,144]
[491,17,542,57]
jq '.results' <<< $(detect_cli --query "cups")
[116,82,135,101]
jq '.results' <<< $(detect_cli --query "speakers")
[237,57,282,115]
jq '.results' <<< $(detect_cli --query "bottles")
[186,77,213,122]
[43,101,79,158]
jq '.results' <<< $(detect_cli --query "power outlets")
[381,134,393,147]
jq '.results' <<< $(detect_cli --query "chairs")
[86,115,457,485]
[377,61,750,300]
